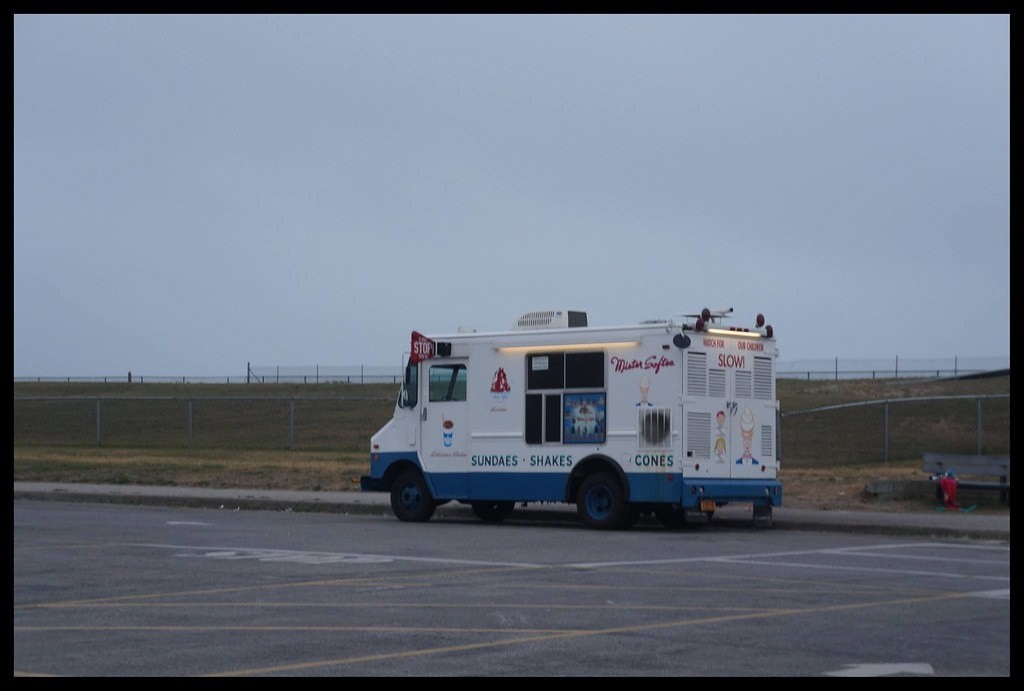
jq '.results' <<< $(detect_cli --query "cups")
[442,420,454,446]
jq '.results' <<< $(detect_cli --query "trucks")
[361,307,783,532]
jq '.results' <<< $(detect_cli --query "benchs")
[922,452,1010,505]
